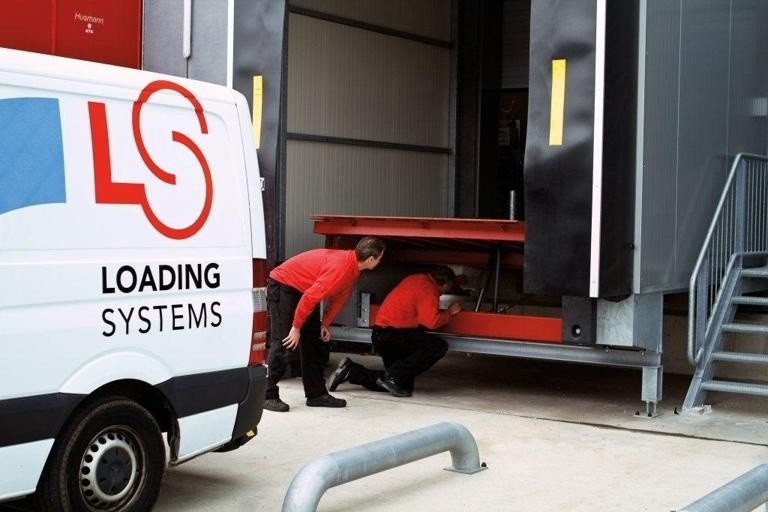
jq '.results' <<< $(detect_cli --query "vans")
[0,47,271,512]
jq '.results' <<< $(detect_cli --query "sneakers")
[264,399,289,411]
[305,393,346,408]
[326,357,353,392]
[375,377,412,396]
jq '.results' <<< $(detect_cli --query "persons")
[327,266,464,398]
[263,236,389,412]
